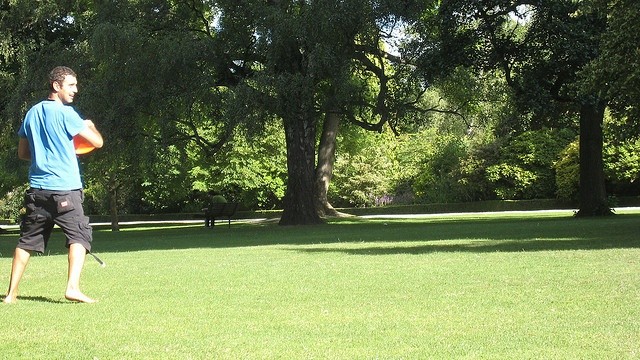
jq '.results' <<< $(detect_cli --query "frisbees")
[73,134,96,155]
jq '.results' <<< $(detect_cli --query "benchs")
[204,202,239,229]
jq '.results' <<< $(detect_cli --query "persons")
[1,64,104,309]
[201,191,229,229]
